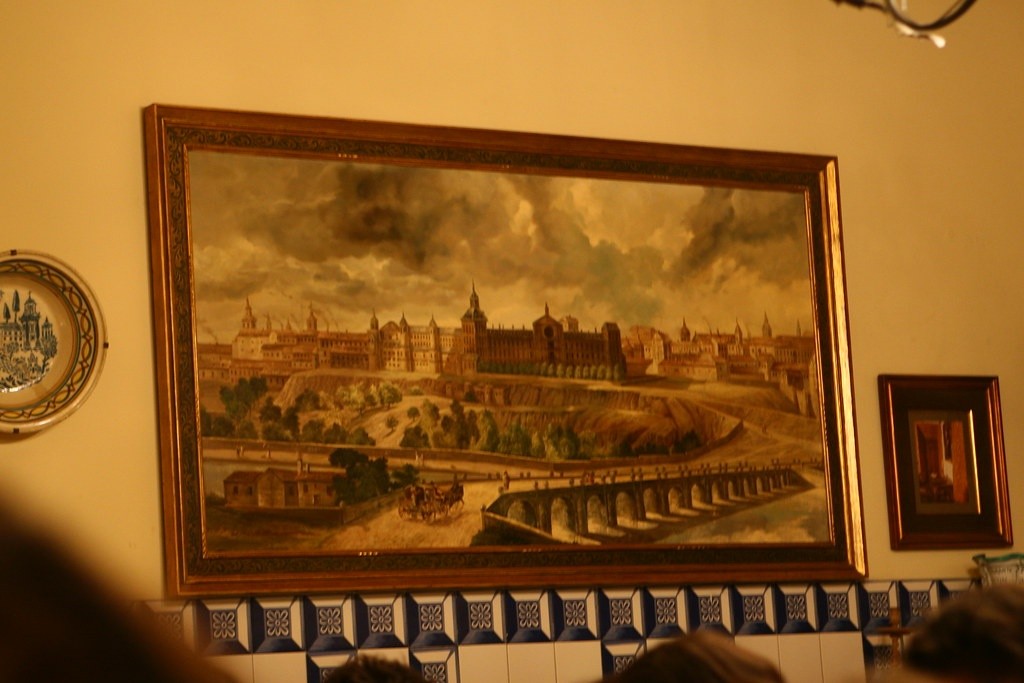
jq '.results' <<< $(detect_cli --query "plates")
[0,249,109,435]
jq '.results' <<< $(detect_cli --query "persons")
[590,627,788,683]
[0,504,239,683]
[895,583,1024,683]
[322,655,427,683]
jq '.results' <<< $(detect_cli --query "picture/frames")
[877,369,1013,552]
[137,100,867,600]
